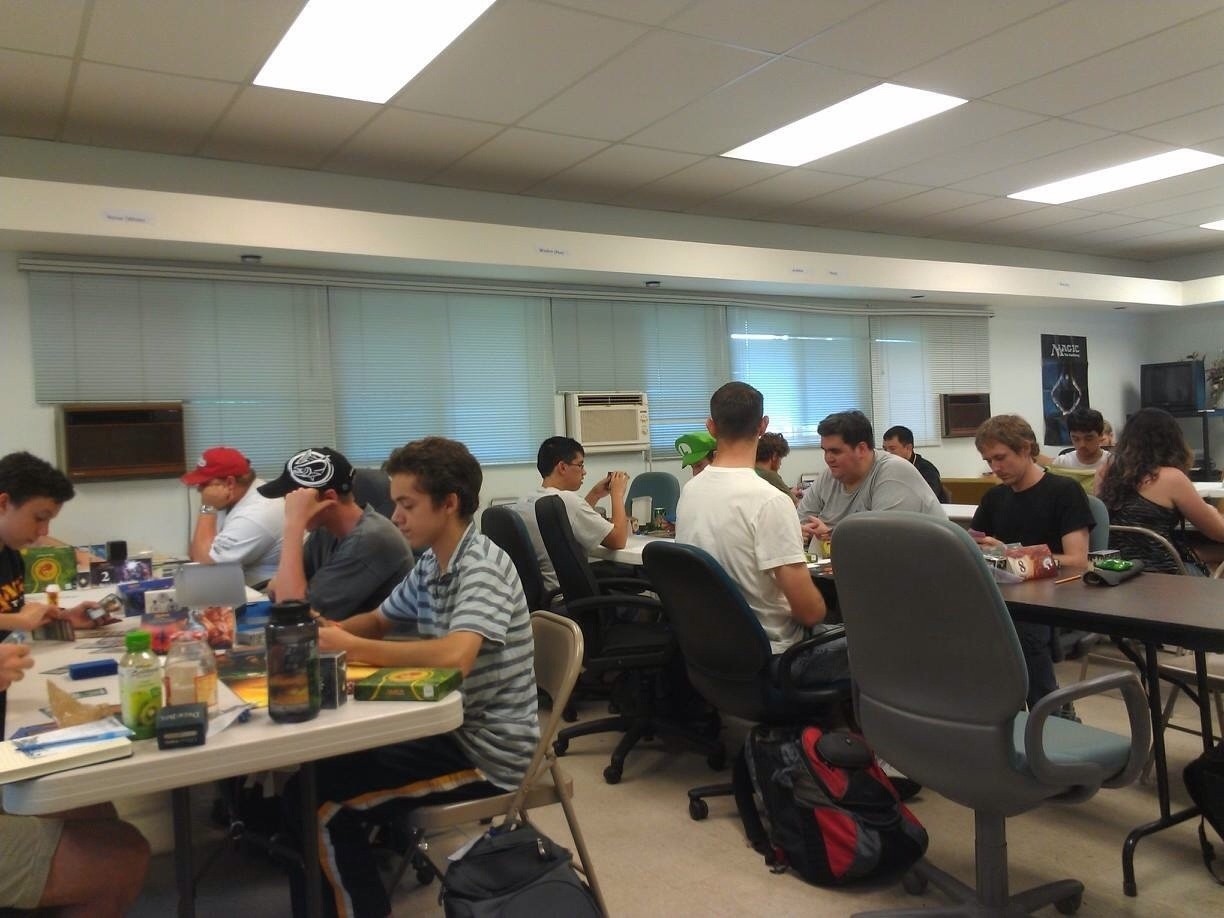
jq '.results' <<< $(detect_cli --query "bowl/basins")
[216,649,268,709]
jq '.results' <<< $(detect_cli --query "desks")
[1125,407,1224,482]
[806,547,1224,898]
[1208,486,1224,499]
[1190,480,1224,500]
[938,498,979,527]
[0,558,465,918]
[587,521,832,770]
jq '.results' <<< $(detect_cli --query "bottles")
[265,599,321,722]
[119,630,161,740]
[165,630,219,720]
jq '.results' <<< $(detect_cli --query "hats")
[256,447,356,499]
[180,447,251,485]
[675,433,716,469]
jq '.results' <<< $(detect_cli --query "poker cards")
[69,688,106,700]
[86,592,123,619]
[76,628,135,654]
[39,706,54,719]
[605,472,613,491]
[39,663,72,674]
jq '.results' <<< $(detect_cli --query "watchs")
[200,503,218,514]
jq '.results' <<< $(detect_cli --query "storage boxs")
[314,646,350,710]
[114,576,175,618]
[153,700,208,752]
[353,665,464,703]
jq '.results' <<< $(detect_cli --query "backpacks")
[438,821,604,918]
[731,721,928,890]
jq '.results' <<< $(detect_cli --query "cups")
[820,539,831,559]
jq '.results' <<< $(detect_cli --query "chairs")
[209,471,1224,918]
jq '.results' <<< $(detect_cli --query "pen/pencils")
[239,710,248,720]
[15,730,131,750]
[1054,575,1081,583]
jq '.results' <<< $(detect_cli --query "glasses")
[559,460,584,469]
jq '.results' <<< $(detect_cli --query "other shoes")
[1062,703,1082,724]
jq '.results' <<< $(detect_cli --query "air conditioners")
[563,390,651,455]
[939,392,993,439]
[52,400,190,484]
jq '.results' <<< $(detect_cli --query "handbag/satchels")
[1171,531,1191,563]
[1183,739,1224,842]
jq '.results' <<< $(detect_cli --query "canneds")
[163,630,218,717]
[653,508,666,529]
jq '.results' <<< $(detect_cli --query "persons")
[0,434,543,918]
[523,383,1224,802]
